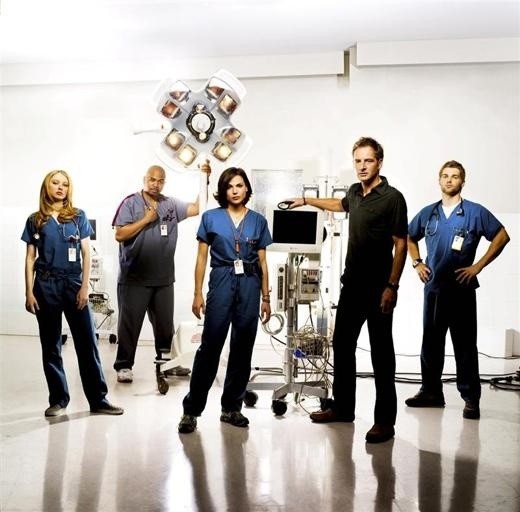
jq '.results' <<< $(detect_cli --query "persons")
[111,158,211,384]
[178,166,273,434]
[18,168,125,418]
[276,136,408,443]
[406,158,513,422]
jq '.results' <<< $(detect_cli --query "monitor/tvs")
[87,217,99,246]
[266,205,325,254]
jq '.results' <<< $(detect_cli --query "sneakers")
[45,404,67,416]
[463,400,480,419]
[366,425,394,443]
[90,403,124,415]
[310,409,355,423]
[220,412,249,426]
[405,391,445,408]
[178,415,197,433]
[117,369,133,382]
[165,366,191,376]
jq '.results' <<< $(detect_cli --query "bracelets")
[303,197,306,205]
[262,298,270,303]
[263,295,270,297]
[387,283,399,291]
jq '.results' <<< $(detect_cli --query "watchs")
[412,258,423,269]
